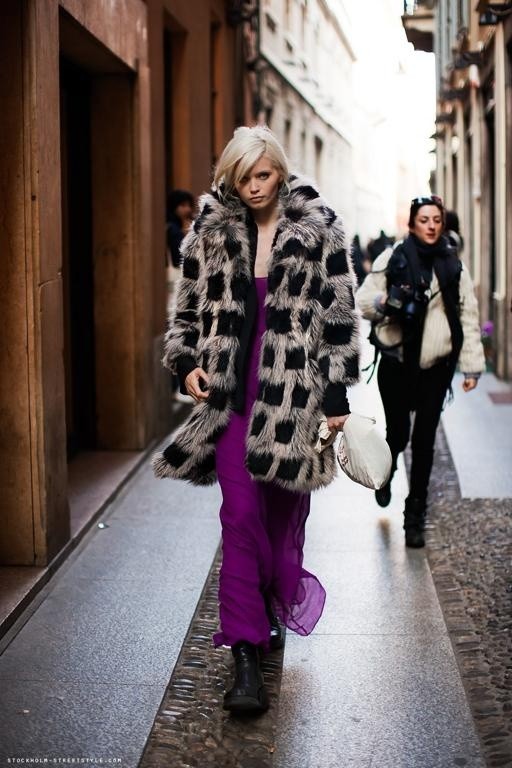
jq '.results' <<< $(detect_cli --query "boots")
[404,497,426,549]
[263,593,281,652]
[374,464,397,508]
[222,641,269,713]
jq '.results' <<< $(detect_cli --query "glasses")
[411,198,434,205]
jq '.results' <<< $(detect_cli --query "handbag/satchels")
[384,285,429,325]
[338,414,393,491]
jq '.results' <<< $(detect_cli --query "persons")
[167,191,197,266]
[353,198,486,549]
[152,125,361,714]
[351,229,394,285]
